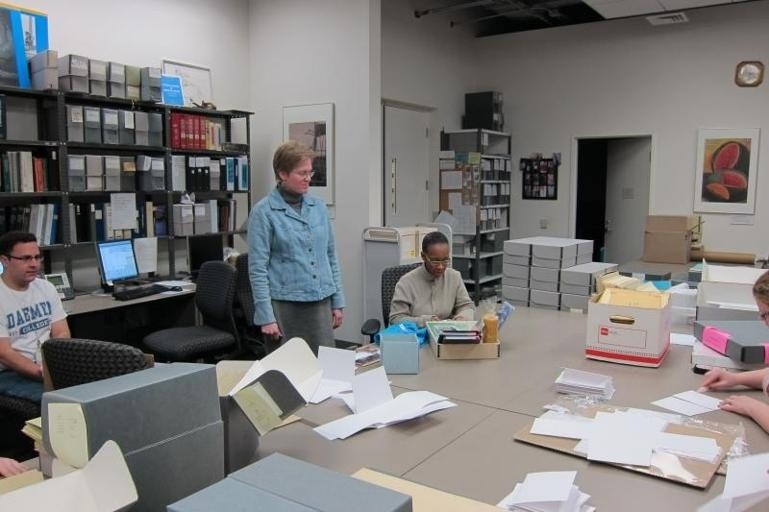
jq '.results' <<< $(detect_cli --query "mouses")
[171,286,183,291]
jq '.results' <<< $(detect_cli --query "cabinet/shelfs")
[438,128,514,297]
[362,221,454,329]
[0,87,253,296]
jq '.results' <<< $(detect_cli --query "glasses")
[289,170,315,178]
[2,253,44,263]
[425,253,450,265]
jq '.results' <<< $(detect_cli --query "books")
[436,331,482,345]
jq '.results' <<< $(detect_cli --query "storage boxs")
[172,203,210,237]
[31,51,167,195]
[1,2,50,87]
[501,234,620,314]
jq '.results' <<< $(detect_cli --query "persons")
[700,268,769,436]
[0,229,72,402]
[247,139,347,357]
[388,231,476,329]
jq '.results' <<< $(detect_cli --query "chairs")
[234,252,274,355]
[145,262,241,358]
[360,260,422,344]
[0,391,40,422]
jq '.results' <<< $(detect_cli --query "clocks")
[733,59,763,88]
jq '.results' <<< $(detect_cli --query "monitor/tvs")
[185,233,224,282]
[95,238,140,287]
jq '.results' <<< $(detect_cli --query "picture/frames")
[691,126,762,216]
[280,101,339,208]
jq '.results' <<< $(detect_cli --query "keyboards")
[112,284,169,302]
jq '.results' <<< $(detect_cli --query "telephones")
[38,272,75,300]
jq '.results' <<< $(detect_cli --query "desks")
[62,277,193,318]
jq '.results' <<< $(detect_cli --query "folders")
[500,159,506,180]
[487,160,493,180]
[496,208,500,228]
[492,209,496,229]
[483,184,488,205]
[488,209,493,229]
[220,158,227,190]
[492,184,497,205]
[506,160,510,180]
[210,159,221,190]
[482,160,487,180]
[499,183,505,204]
[505,183,510,204]
[480,209,488,230]
[203,157,210,190]
[185,156,196,191]
[493,159,500,180]
[487,184,492,205]
[196,157,203,191]
